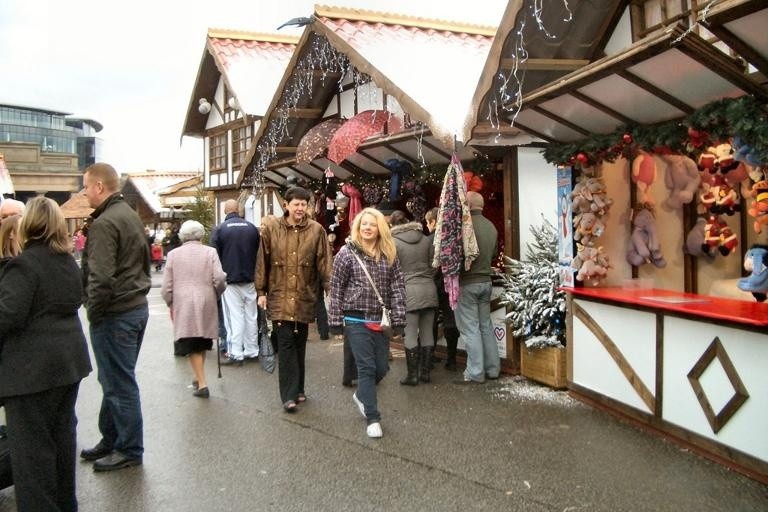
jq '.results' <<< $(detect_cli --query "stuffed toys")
[698,142,767,299]
[627,156,700,272]
[566,171,615,283]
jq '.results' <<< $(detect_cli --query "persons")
[0,198,26,221]
[329,207,406,437]
[74,216,182,277]
[209,199,264,366]
[447,189,504,384]
[81,165,152,473]
[253,187,336,411]
[386,209,432,386]
[160,220,227,399]
[0,197,93,512]
[422,205,459,378]
[0,215,27,259]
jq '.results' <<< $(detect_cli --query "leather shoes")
[218,357,243,367]
[93,452,142,471]
[193,387,210,398]
[298,393,306,402]
[80,447,108,459]
[283,400,297,410]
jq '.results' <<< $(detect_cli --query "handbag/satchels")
[380,308,394,338]
[259,319,276,374]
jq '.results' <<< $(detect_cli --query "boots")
[400,326,475,385]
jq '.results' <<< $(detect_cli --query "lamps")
[228,97,236,109]
[198,92,211,115]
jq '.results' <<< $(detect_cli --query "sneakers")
[366,422,384,438]
[352,393,368,418]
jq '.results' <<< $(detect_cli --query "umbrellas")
[324,110,393,167]
[294,120,345,163]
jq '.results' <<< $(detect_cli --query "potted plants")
[491,213,567,390]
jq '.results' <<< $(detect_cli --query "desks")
[555,286,768,486]
